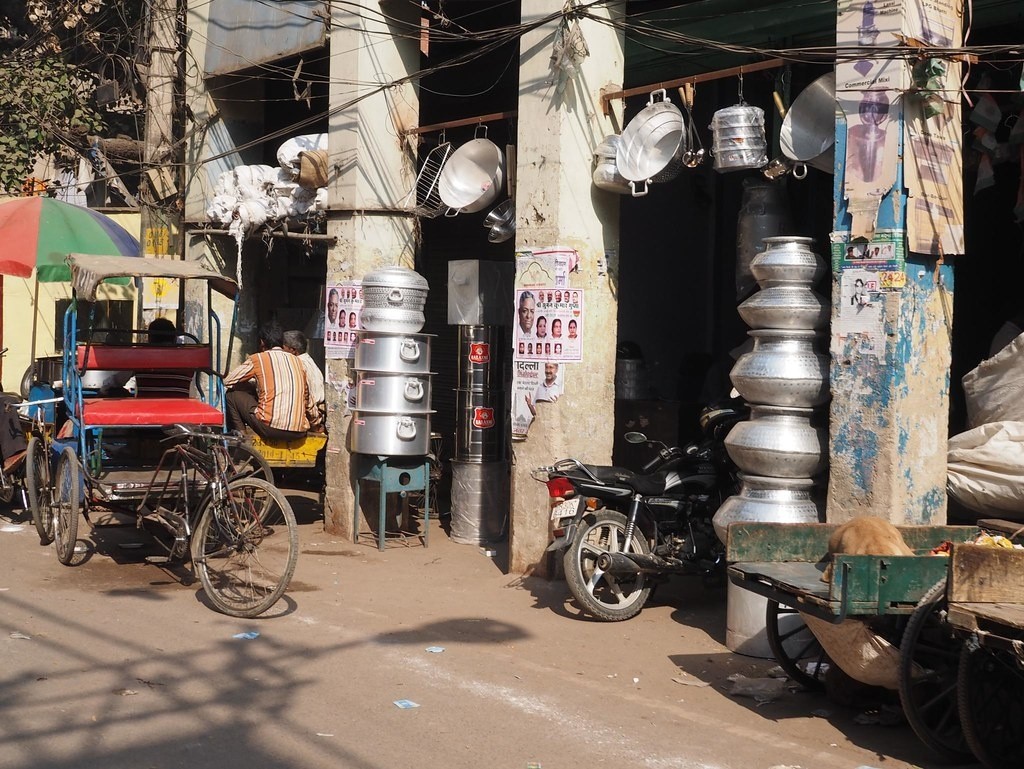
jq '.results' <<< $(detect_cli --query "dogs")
[821,515,916,583]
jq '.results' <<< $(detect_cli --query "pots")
[350,407,438,455]
[350,328,439,372]
[707,104,768,173]
[615,89,687,197]
[592,130,645,195]
[350,368,439,412]
[439,125,506,217]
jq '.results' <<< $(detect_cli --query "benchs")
[74,340,225,430]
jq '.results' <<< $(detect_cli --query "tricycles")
[46,252,301,618]
[726,521,1024,761]
[943,539,1024,769]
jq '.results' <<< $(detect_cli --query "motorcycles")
[530,387,742,623]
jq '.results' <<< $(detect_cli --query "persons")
[844,243,895,260]
[326,289,364,342]
[279,328,327,434]
[517,290,580,356]
[524,360,564,417]
[94,317,195,469]
[850,278,868,306]
[220,320,310,443]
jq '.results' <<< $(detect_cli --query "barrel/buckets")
[449,458,509,544]
[452,388,506,461]
[456,323,504,389]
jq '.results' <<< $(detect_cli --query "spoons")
[759,90,794,183]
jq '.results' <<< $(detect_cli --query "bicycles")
[0,395,65,541]
[226,439,274,536]
